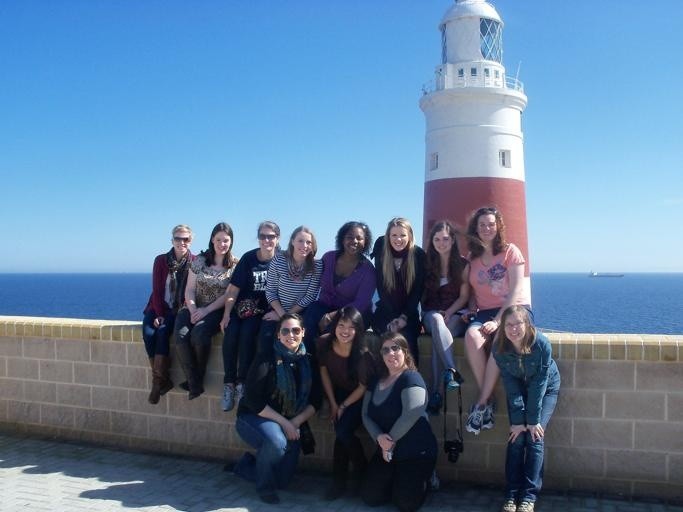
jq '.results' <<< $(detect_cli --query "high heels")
[224,453,249,473]
[259,496,279,505]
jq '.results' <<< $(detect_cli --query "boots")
[178,348,206,393]
[347,454,358,497]
[175,344,199,400]
[328,441,349,500]
[149,357,174,395]
[148,354,166,404]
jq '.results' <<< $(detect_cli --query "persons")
[424,218,469,416]
[362,331,441,511]
[140,224,197,404]
[262,224,322,322]
[220,221,284,413]
[223,311,324,506]
[463,205,524,436]
[319,220,376,332]
[374,217,424,372]
[491,304,561,512]
[174,222,239,401]
[314,304,374,500]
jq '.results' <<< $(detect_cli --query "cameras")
[444,438,463,463]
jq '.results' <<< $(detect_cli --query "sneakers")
[426,395,440,416]
[444,368,465,392]
[501,499,516,512]
[233,382,245,405]
[430,469,440,491]
[516,502,534,512]
[220,384,234,411]
[481,399,496,430]
[464,404,485,436]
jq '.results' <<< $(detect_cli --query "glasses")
[380,344,401,355]
[257,234,275,240]
[504,319,526,327]
[279,328,299,336]
[173,236,190,242]
